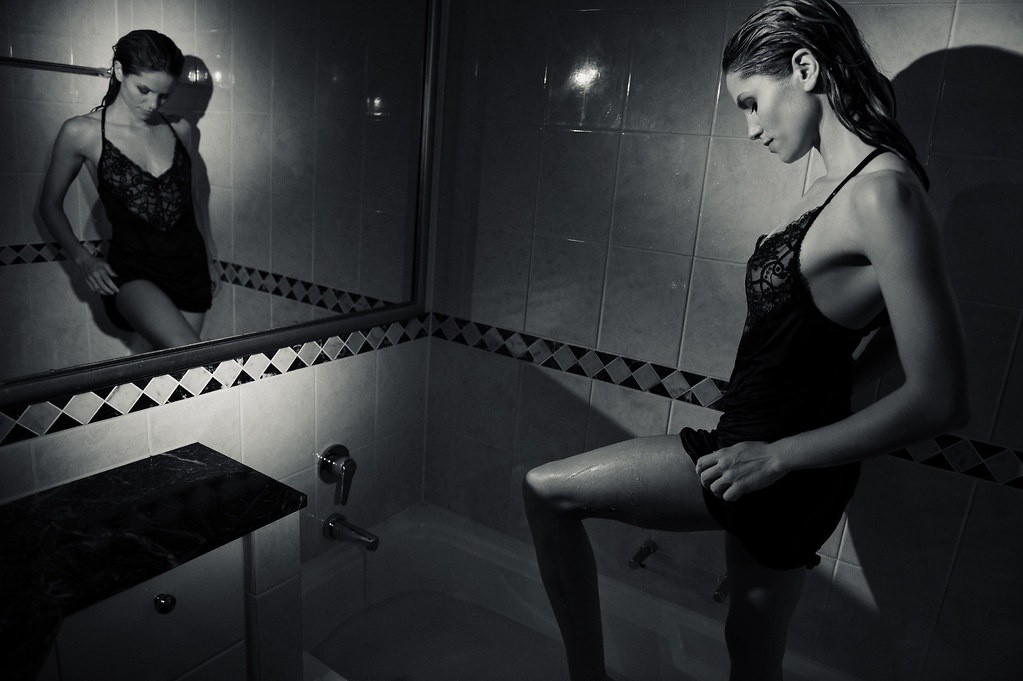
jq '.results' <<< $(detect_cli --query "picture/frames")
[0,1,442,407]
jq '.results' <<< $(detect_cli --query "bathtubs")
[294,500,857,681]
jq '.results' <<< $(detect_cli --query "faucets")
[323,512,380,552]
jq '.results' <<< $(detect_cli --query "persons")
[521,0,972,681]
[39,29,221,346]
